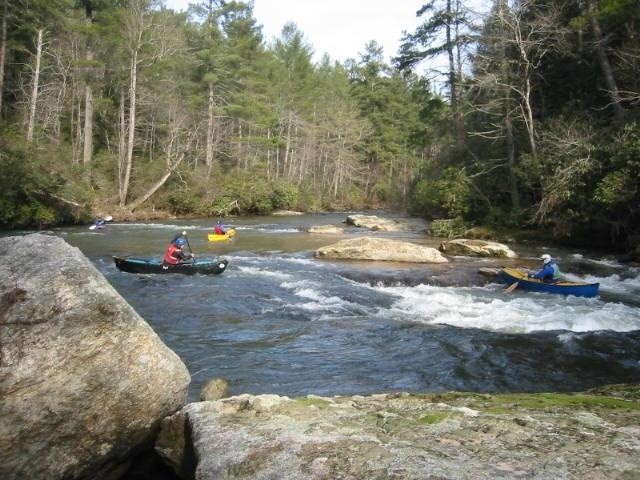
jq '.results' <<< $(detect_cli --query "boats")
[208,229,236,244]
[112,254,228,275]
[89,225,106,231]
[502,266,601,299]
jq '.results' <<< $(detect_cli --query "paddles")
[504,259,541,295]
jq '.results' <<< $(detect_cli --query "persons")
[96,217,104,225]
[528,253,561,282]
[162,230,194,265]
[214,220,225,234]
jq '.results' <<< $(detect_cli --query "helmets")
[175,238,185,244]
[541,254,551,264]
